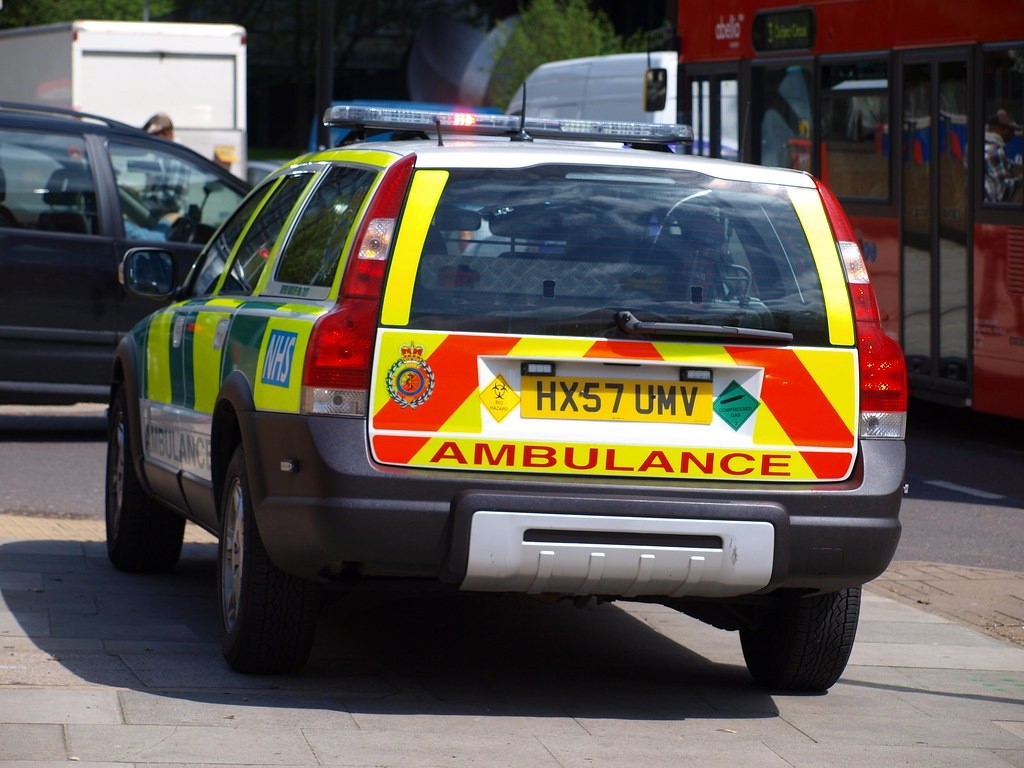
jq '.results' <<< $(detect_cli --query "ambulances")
[105,103,908,695]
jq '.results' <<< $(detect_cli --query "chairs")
[37,168,87,235]
[487,185,572,261]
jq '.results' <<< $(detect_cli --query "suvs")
[0,99,257,406]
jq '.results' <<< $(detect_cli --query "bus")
[643,3,1023,424]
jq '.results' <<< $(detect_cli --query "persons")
[142,113,191,209]
[81,166,182,241]
[964,108,1024,203]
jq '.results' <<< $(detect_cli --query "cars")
[247,156,290,191]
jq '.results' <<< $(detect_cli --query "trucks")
[1,17,247,186]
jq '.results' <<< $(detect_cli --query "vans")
[504,47,736,158]
